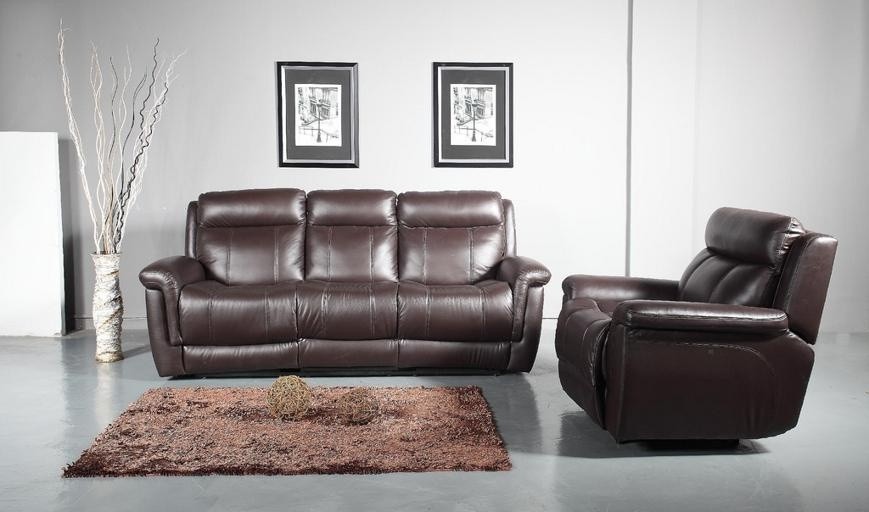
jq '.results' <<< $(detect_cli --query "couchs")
[139,188,551,376]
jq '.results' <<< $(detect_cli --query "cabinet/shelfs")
[0,131,78,336]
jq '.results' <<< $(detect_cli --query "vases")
[90,253,124,363]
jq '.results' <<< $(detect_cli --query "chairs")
[555,206,839,452]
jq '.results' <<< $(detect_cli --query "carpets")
[64,387,516,477]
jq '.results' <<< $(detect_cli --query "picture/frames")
[433,61,513,168]
[276,61,360,170]
